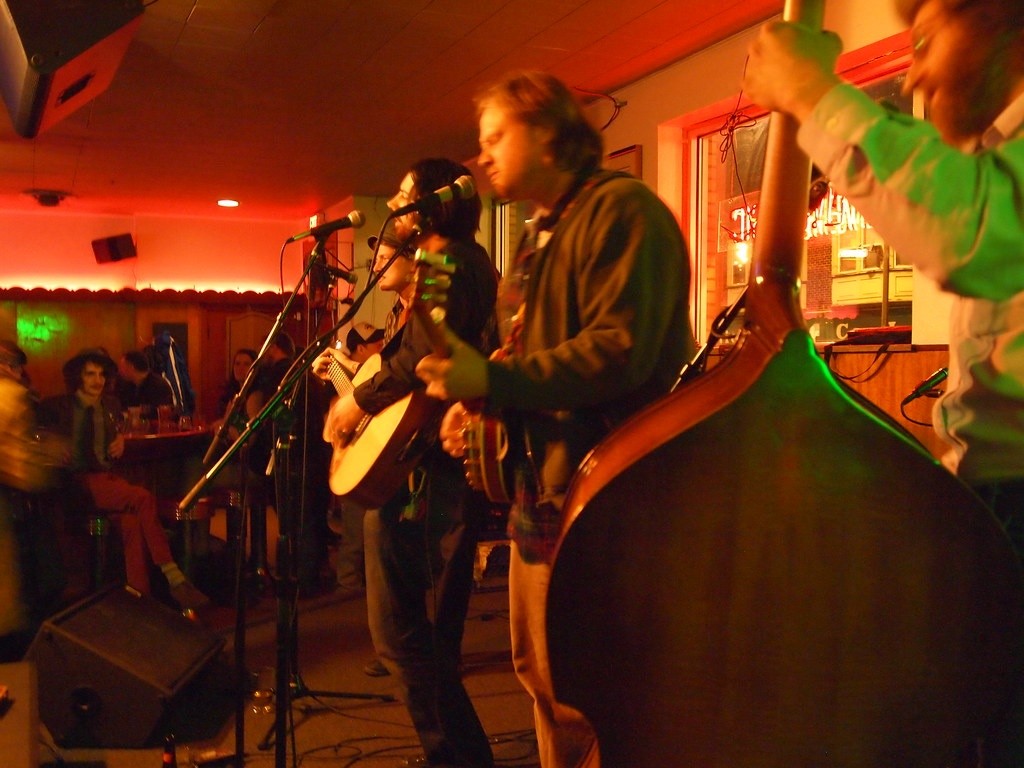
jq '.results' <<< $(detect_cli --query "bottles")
[161,734,177,768]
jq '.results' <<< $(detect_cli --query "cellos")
[541,0,1024,768]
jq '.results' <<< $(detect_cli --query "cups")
[179,416,191,431]
[157,404,176,432]
[128,405,150,434]
[251,666,273,715]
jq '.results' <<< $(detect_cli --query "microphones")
[392,176,477,218]
[314,260,359,283]
[287,209,366,243]
[901,367,949,407]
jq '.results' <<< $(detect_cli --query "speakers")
[0,0,147,138]
[23,580,228,750]
[91,233,136,264]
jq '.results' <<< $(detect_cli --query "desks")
[125,427,211,443]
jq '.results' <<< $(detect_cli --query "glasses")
[912,3,966,58]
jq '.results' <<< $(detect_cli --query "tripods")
[256,257,396,753]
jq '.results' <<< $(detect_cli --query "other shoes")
[365,655,392,678]
[169,578,211,610]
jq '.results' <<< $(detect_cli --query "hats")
[368,228,414,260]
[62,346,119,387]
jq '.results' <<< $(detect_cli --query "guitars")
[412,246,518,508]
[315,349,438,512]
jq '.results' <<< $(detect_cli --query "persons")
[0,338,78,654]
[416,71,702,768]
[736,2,1024,566]
[35,347,211,611]
[108,331,333,595]
[307,158,503,768]
[326,322,387,600]
[366,226,419,677]
